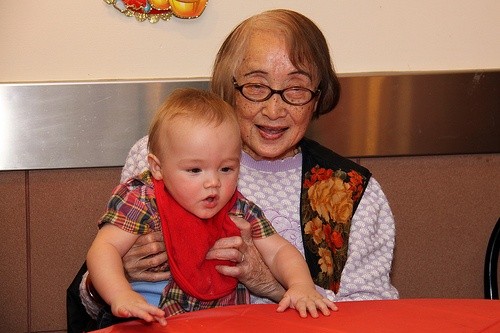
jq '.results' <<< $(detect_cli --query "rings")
[241,254,244,262]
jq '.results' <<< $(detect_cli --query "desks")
[86,297,500,333]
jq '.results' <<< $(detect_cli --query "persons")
[66,7,400,333]
[85,87,339,327]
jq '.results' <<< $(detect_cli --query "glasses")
[232,75,323,106]
